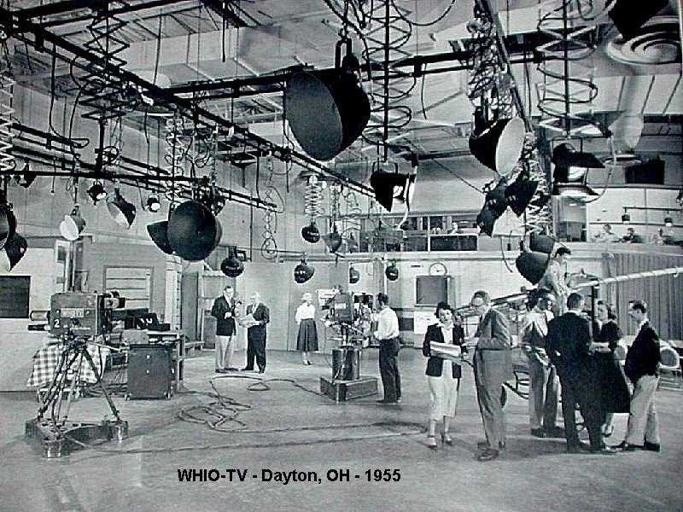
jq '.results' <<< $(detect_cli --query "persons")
[431,223,441,233]
[447,221,463,234]
[611,299,661,452]
[520,291,630,455]
[211,286,241,372]
[591,221,683,245]
[462,291,513,462]
[422,302,466,447]
[241,292,268,374]
[368,292,401,405]
[538,247,571,316]
[294,293,317,365]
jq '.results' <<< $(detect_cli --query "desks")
[126,337,186,400]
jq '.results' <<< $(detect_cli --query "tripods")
[24,339,125,437]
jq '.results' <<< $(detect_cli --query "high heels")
[440,430,453,444]
[428,434,437,449]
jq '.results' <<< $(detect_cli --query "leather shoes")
[479,449,498,460]
[478,441,505,449]
[531,427,660,455]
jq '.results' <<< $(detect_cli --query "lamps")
[0,0,607,285]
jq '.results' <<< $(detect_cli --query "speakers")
[128,344,173,399]
